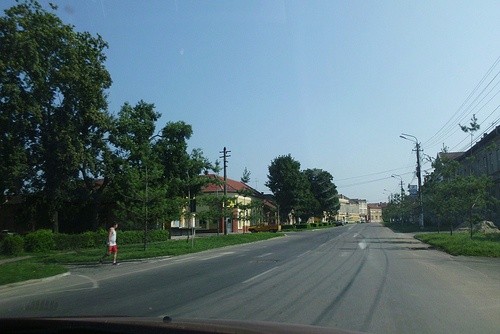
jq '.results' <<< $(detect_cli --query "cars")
[335,220,345,226]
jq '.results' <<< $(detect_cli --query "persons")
[99,223,119,264]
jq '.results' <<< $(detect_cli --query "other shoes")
[113,262,119,265]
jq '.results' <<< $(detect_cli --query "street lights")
[400,132,424,231]
